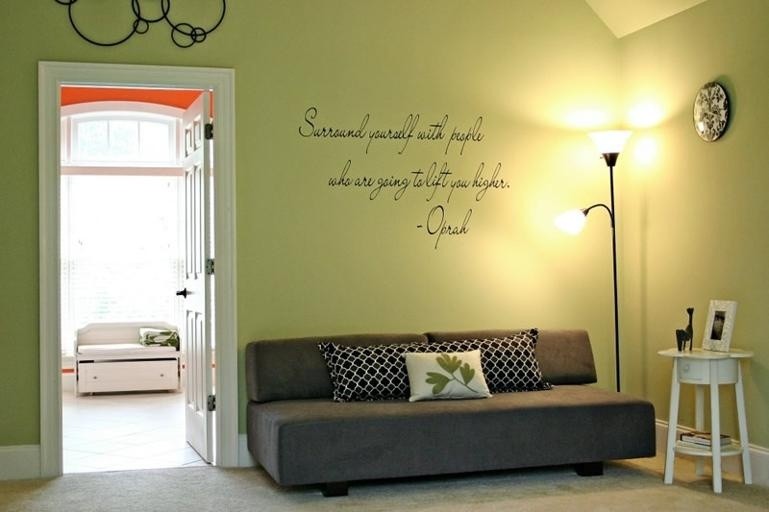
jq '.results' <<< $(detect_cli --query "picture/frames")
[702,299,738,352]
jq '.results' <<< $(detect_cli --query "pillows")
[318,329,551,401]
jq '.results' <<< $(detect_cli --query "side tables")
[656,348,754,493]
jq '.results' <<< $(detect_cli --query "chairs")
[75,321,179,353]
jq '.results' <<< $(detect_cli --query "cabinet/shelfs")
[75,353,178,396]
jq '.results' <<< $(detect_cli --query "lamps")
[539,130,632,392]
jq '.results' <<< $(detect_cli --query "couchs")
[244,328,652,499]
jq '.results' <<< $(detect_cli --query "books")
[679,430,731,447]
